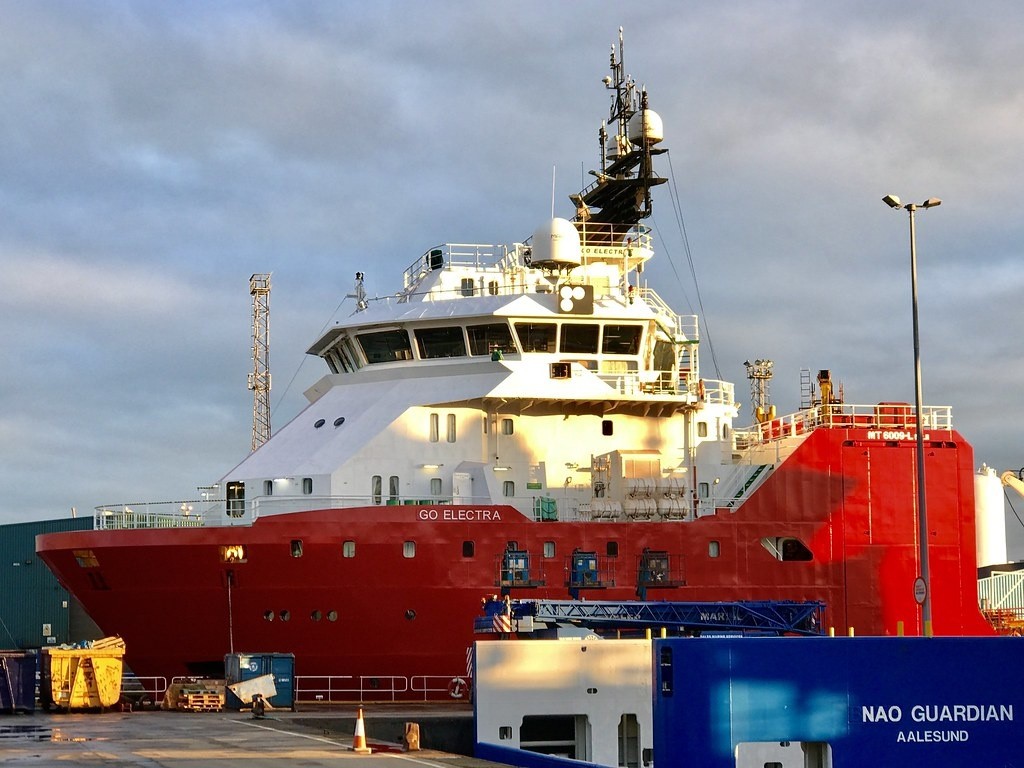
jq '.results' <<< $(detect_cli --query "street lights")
[880,194,942,636]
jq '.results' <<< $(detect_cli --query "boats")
[32,27,1022,712]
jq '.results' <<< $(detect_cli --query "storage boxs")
[224,653,295,709]
[0,514,204,651]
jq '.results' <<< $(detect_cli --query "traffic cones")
[347,709,372,754]
